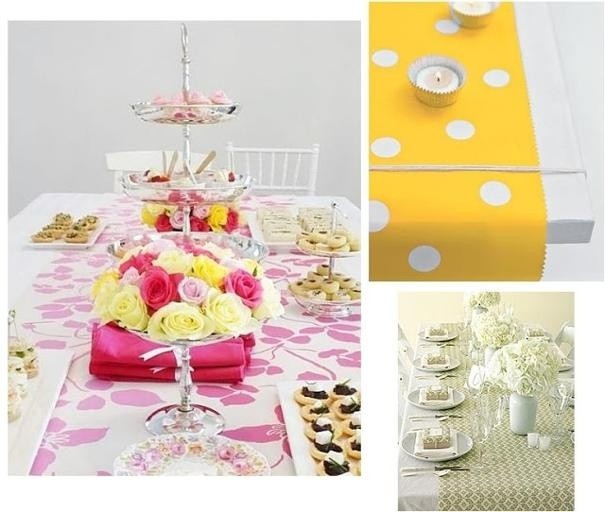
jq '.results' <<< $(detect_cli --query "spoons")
[400,468,452,477]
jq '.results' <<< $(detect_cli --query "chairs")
[226,141,320,195]
[105,150,212,192]
[398,326,415,367]
[554,320,575,358]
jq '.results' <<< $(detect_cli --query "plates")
[417,328,458,342]
[8,345,75,476]
[24,217,112,251]
[108,432,273,475]
[406,386,466,410]
[399,427,476,464]
[245,208,355,252]
[272,380,360,476]
[412,355,461,373]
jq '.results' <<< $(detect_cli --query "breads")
[289,263,361,301]
[30,213,99,243]
[296,379,361,476]
[295,230,361,253]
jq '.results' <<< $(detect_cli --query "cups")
[525,430,553,453]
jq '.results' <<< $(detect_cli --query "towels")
[412,428,457,458]
[86,322,257,383]
[419,389,454,408]
[425,327,449,340]
[422,355,451,370]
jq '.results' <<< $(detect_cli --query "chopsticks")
[402,465,470,470]
[404,412,464,419]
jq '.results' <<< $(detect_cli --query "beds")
[399,320,574,510]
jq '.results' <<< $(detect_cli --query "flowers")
[461,292,563,397]
[91,242,286,340]
[141,197,244,233]
